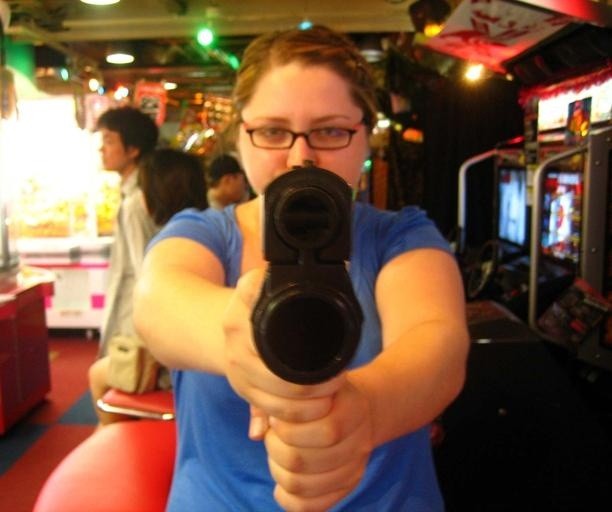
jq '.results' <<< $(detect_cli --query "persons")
[131,25,472,512]
[204,152,248,213]
[87,147,209,432]
[88,105,159,427]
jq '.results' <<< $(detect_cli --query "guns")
[249,159,364,385]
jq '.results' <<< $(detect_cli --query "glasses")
[239,119,364,149]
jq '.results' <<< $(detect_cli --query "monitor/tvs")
[540,172,583,263]
[498,169,526,246]
